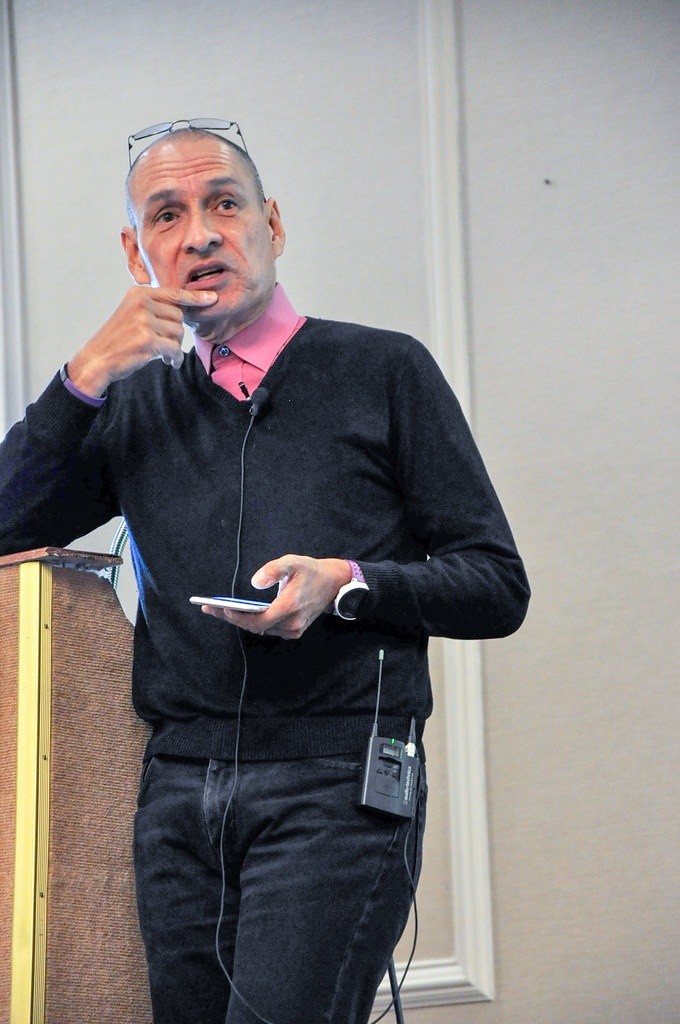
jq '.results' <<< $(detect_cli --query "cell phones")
[190,597,270,613]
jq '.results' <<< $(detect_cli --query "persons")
[0,118,531,1024]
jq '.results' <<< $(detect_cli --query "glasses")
[128,119,247,169]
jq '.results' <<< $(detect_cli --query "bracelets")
[60,363,108,407]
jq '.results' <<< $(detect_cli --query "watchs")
[332,559,370,620]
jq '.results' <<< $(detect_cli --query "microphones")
[251,387,269,416]
